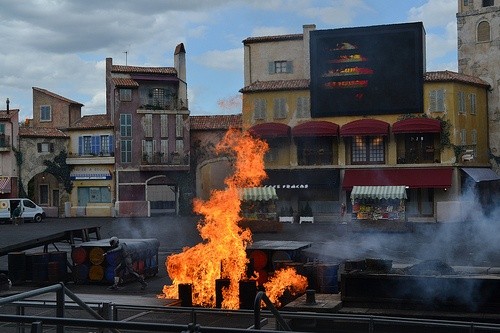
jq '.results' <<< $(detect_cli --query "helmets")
[110,237,119,248]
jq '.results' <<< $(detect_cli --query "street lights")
[122,52,127,66]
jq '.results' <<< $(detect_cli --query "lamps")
[42,172,45,180]
[108,184,115,192]
[488,147,497,154]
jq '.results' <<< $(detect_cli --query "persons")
[12,205,21,225]
[102,237,148,291]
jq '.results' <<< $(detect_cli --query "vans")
[0,199,47,223]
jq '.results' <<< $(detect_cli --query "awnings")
[245,122,291,138]
[340,119,389,135]
[342,169,452,188]
[350,185,408,200]
[292,121,338,137]
[0,176,11,194]
[392,119,440,132]
[460,167,500,183]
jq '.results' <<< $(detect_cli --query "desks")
[300,217,314,224]
[279,217,294,224]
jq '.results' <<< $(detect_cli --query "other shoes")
[109,286,120,291]
[141,283,148,290]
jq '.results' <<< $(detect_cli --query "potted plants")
[142,152,189,165]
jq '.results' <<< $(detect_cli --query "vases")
[65,202,71,217]
